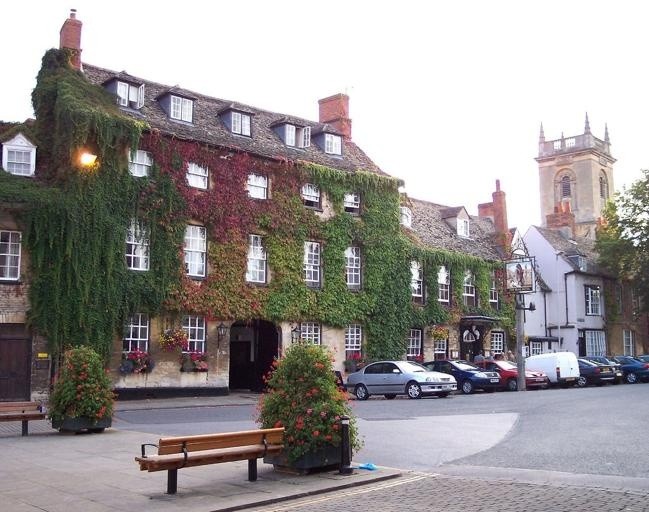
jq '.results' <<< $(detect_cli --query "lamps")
[217,323,228,348]
[516,301,536,311]
[291,326,300,343]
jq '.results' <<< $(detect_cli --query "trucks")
[523,352,582,389]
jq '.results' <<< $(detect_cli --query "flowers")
[120,350,154,374]
[158,329,188,353]
[180,351,209,371]
[43,344,117,425]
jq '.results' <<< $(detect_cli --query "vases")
[262,420,353,475]
[61,413,112,434]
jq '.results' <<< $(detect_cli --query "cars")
[342,357,547,403]
[576,355,649,388]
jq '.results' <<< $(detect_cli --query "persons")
[473,350,515,362]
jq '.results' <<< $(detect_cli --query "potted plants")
[253,338,365,457]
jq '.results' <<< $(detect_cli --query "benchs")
[0,401,45,434]
[135,428,286,494]
